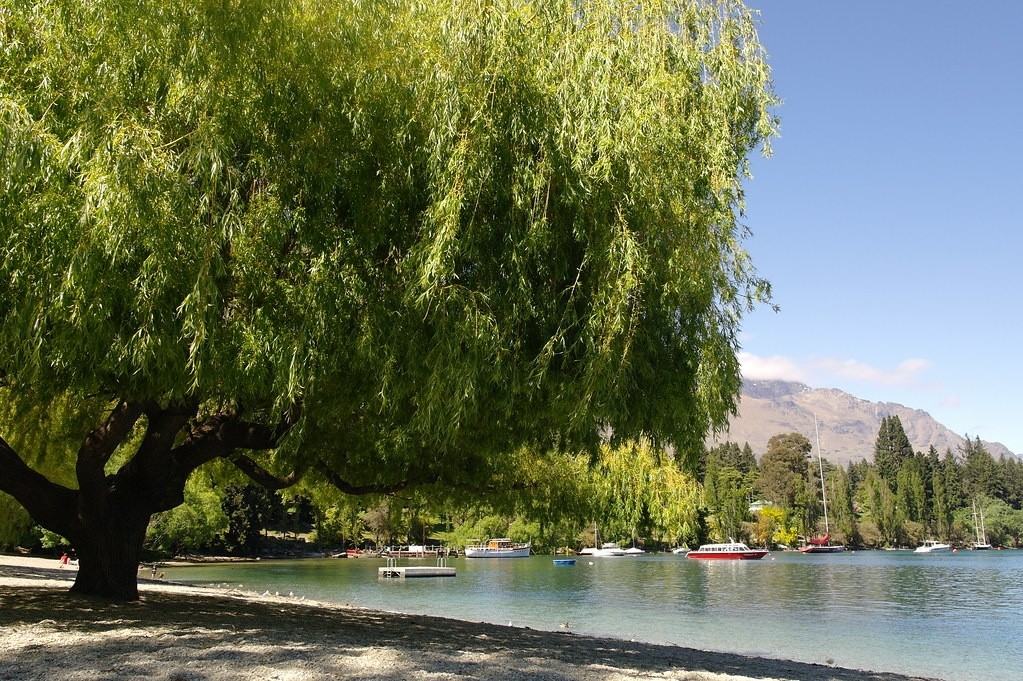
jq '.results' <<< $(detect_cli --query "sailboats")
[966,500,993,550]
[576,520,646,557]
[345,533,395,559]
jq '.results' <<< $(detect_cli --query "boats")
[774,535,846,554]
[465,517,533,558]
[912,539,952,554]
[553,559,577,566]
[684,536,770,560]
[672,543,692,554]
[377,554,456,578]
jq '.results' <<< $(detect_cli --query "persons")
[61,553,68,564]
